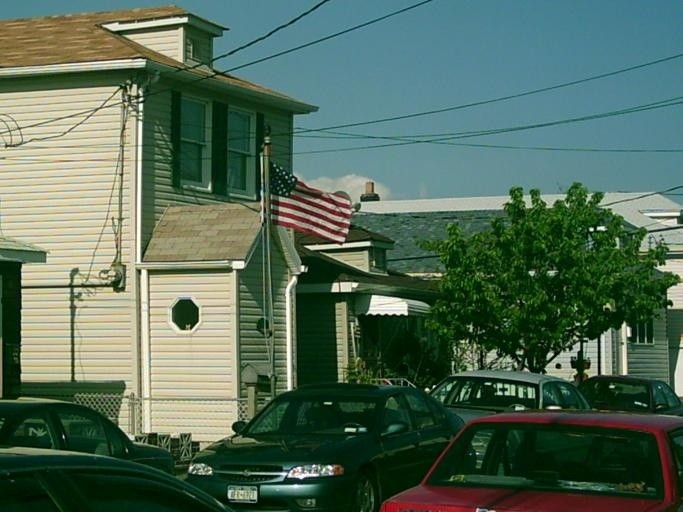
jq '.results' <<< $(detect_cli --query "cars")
[423,370,593,472]
[380,412,683,512]
[0,396,177,478]
[0,445,236,511]
[187,383,476,512]
[571,376,683,421]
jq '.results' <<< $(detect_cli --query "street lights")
[594,297,615,375]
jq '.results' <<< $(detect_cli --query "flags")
[259,153,353,246]
